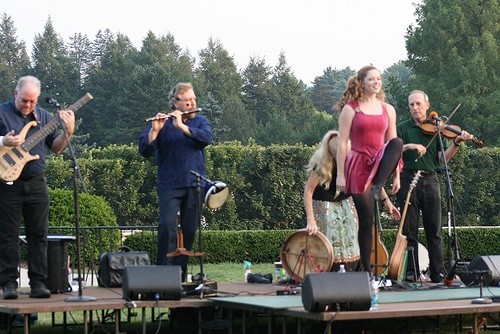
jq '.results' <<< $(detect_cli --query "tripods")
[182,170,239,299]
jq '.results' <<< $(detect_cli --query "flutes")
[145,108,202,122]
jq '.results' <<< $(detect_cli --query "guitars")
[344,225,388,276]
[0,92,94,184]
[387,169,422,280]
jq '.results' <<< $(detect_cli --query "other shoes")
[431,277,443,283]
[406,274,416,282]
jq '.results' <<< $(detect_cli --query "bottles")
[338,264,346,273]
[369,281,379,311]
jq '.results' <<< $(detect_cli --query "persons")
[0,76,75,300]
[396,89,471,290]
[139,82,211,284]
[335,66,404,272]
[303,130,400,272]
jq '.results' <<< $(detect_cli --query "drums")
[282,228,334,283]
[204,180,228,209]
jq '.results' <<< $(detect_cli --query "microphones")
[45,97,60,107]
[212,185,229,194]
[444,260,458,286]
[429,112,441,121]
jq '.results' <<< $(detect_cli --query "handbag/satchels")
[98,246,150,288]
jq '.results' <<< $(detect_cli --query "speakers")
[98,253,150,287]
[301,271,372,312]
[122,265,182,301]
[460,255,500,286]
[45,240,69,294]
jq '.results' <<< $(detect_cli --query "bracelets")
[382,197,389,201]
[454,140,460,146]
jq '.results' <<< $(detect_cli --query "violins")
[418,118,484,147]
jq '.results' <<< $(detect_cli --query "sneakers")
[3,283,18,299]
[369,280,378,304]
[29,281,51,298]
